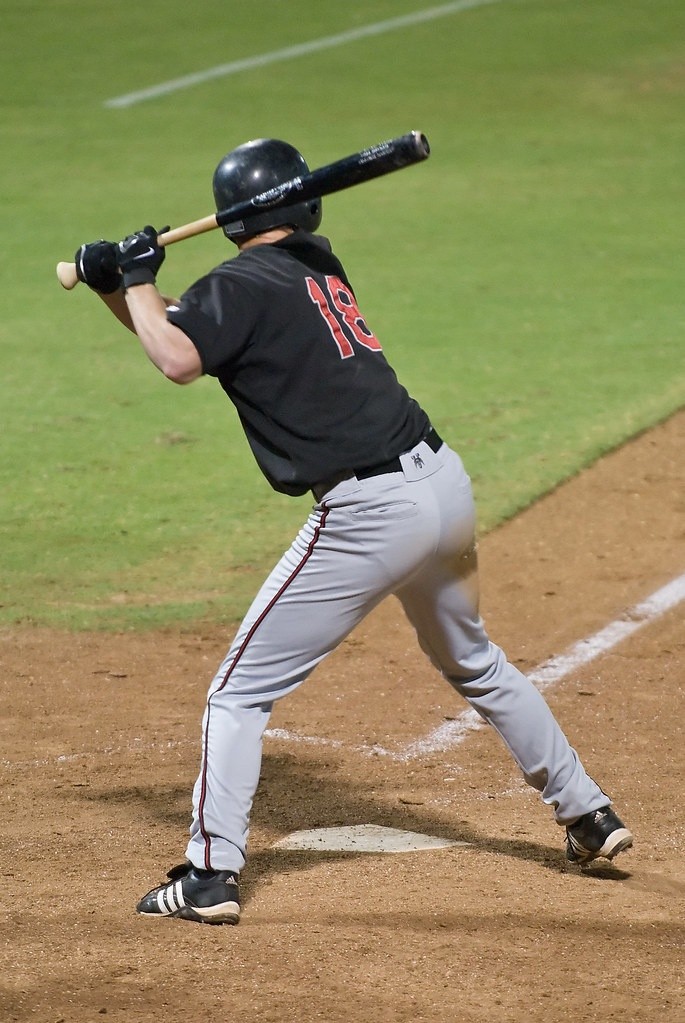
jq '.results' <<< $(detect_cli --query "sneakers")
[136,863,241,925]
[564,806,633,865]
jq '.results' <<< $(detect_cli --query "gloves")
[75,241,118,294]
[115,225,171,288]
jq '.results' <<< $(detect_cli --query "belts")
[352,427,443,481]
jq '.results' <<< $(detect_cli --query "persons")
[77,137,635,927]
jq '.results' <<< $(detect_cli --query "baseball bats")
[53,127,432,292]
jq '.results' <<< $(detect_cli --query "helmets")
[213,138,323,244]
